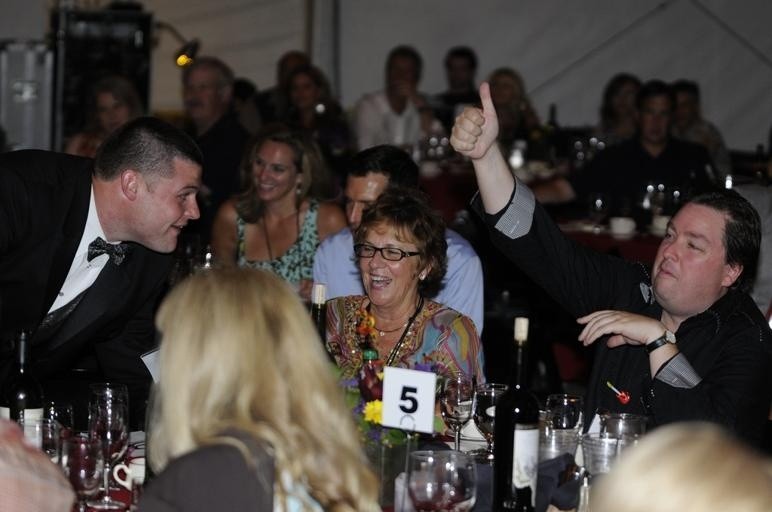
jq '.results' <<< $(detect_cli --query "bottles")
[305,282,335,375]
[0,330,44,448]
[639,181,682,241]
[494,317,539,512]
[196,241,219,270]
[751,142,767,183]
[545,103,561,132]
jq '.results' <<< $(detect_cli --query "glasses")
[353,243,422,262]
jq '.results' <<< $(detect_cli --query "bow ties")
[86,236,129,266]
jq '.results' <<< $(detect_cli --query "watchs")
[647,330,679,353]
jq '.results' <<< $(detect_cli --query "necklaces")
[373,324,408,337]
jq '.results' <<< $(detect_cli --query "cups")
[544,394,583,455]
[611,217,636,233]
[581,432,626,482]
[405,449,478,512]
[601,412,644,438]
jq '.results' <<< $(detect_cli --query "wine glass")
[18,382,128,511]
[441,371,478,452]
[471,384,510,465]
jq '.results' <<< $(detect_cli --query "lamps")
[155,20,199,65]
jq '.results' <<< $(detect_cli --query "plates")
[607,232,638,241]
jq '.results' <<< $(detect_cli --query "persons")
[449,78,772,457]
[312,140,486,342]
[489,67,728,212]
[212,127,347,296]
[180,43,483,200]
[66,77,145,159]
[321,186,489,435]
[122,263,389,511]
[0,116,205,432]
